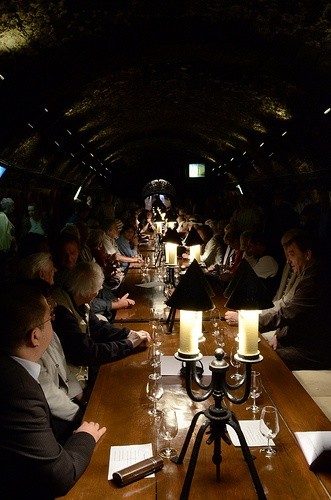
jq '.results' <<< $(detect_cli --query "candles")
[179,309,203,353]
[156,222,163,233]
[190,244,200,264]
[238,309,259,356]
[165,242,179,265]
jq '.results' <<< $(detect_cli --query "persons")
[0,198,18,251]
[21,202,55,237]
[177,209,282,310]
[0,230,151,500]
[151,195,167,211]
[93,207,157,286]
[224,228,331,366]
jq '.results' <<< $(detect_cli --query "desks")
[54,233,331,500]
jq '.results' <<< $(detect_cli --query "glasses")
[24,314,55,342]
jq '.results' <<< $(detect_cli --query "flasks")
[112,455,165,487]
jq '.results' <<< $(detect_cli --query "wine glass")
[147,305,165,376]
[260,405,280,455]
[228,346,244,381]
[145,373,164,417]
[246,371,262,412]
[139,224,173,295]
[210,309,221,336]
[157,408,179,460]
[215,326,228,357]
